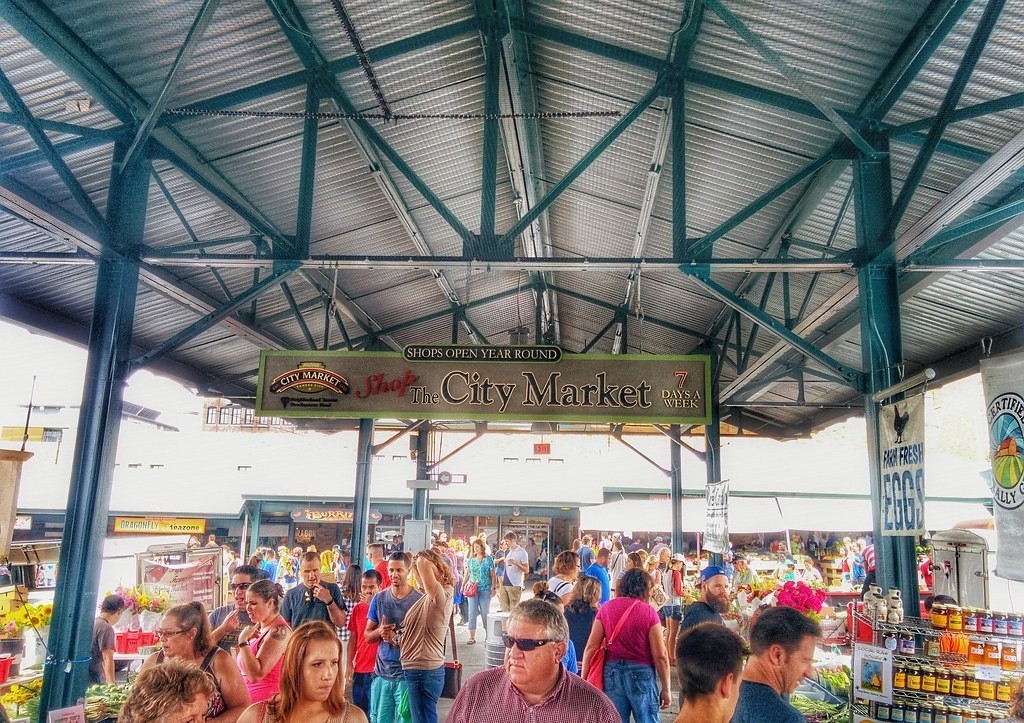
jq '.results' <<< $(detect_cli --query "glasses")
[155,628,188,638]
[502,632,561,651]
[545,592,556,604]
[305,590,311,605]
[229,582,252,591]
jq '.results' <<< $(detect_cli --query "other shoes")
[457,617,468,625]
[476,609,480,616]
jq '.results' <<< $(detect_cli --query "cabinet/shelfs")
[848,600,1024,723]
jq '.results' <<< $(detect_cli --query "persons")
[399,550,455,723]
[445,599,622,723]
[926,595,967,669]
[190,531,403,696]
[921,550,933,590]
[235,621,369,723]
[345,569,383,723]
[673,623,749,723]
[87,595,124,685]
[581,567,671,723]
[140,602,252,723]
[432,528,539,645]
[533,532,731,703]
[364,551,423,723]
[722,531,873,601]
[117,662,216,723]
[728,606,822,723]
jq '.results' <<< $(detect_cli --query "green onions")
[21,695,105,723]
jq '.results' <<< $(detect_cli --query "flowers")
[105,587,175,613]
[2,600,53,637]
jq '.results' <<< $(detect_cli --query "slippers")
[467,638,475,645]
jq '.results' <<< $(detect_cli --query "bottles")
[863,584,903,624]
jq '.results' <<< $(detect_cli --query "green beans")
[786,694,841,719]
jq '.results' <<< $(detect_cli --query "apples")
[89,682,128,701]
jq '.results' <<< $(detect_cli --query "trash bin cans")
[485,613,510,669]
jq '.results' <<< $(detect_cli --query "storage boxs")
[116,632,161,656]
[1,585,31,640]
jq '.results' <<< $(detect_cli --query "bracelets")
[238,641,249,647]
[325,598,334,606]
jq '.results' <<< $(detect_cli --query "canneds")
[874,603,1024,723]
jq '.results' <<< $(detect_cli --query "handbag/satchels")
[440,662,463,699]
[463,582,478,597]
[586,644,608,691]
[671,611,682,621]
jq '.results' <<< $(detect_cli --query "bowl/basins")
[137,646,155,654]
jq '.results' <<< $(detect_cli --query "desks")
[110,652,156,684]
[1,667,45,717]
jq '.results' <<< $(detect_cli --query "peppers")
[823,664,851,689]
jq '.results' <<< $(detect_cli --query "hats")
[729,554,746,563]
[701,565,726,582]
[671,553,687,565]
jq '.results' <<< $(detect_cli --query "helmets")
[653,536,662,543]
